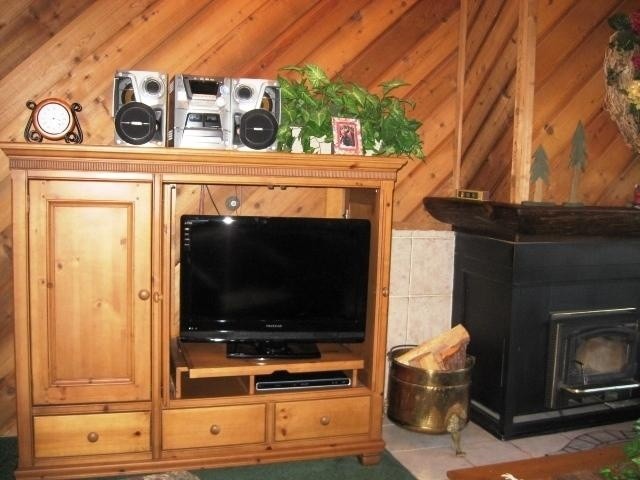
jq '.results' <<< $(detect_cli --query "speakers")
[111,69,170,147]
[231,77,282,152]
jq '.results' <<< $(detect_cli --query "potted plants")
[276,70,425,162]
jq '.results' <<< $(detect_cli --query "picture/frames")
[331,116,363,155]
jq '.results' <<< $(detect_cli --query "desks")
[446,444,640,479]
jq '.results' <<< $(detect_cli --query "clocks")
[24,98,84,145]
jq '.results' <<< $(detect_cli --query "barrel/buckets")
[387,345,476,456]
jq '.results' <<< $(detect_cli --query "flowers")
[602,10,640,156]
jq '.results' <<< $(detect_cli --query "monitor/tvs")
[179,213,372,360]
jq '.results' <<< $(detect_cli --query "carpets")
[0,435,418,480]
[561,430,638,453]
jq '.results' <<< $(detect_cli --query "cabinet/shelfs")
[158,395,374,454]
[11,167,151,467]
